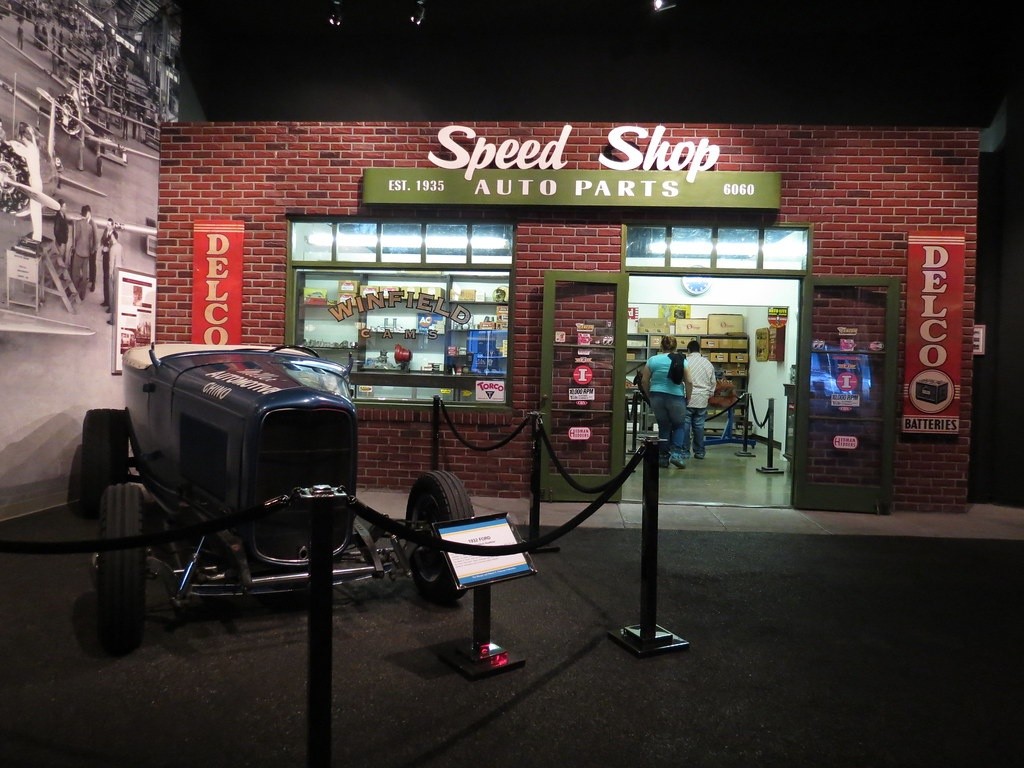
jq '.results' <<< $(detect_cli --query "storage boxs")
[626,313,749,416]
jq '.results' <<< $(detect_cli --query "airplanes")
[0,1,166,244]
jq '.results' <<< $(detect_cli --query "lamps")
[410,5,427,26]
[654,1,677,12]
[328,7,344,27]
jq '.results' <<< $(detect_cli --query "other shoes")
[694,452,705,458]
[90,284,95,292]
[670,452,686,468]
[106,308,110,313]
[681,450,690,459]
[107,320,113,325]
[659,453,669,468]
[100,302,108,306]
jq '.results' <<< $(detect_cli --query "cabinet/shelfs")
[623,331,751,438]
[293,269,511,405]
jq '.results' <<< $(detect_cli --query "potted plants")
[707,378,736,406]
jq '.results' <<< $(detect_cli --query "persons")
[56,200,123,325]
[683,341,716,458]
[9,126,43,242]
[52,25,56,42]
[59,30,63,42]
[42,25,48,35]
[17,25,24,50]
[34,22,39,33]
[0,119,6,141]
[642,336,692,469]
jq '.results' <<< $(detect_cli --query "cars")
[79,338,479,659]
